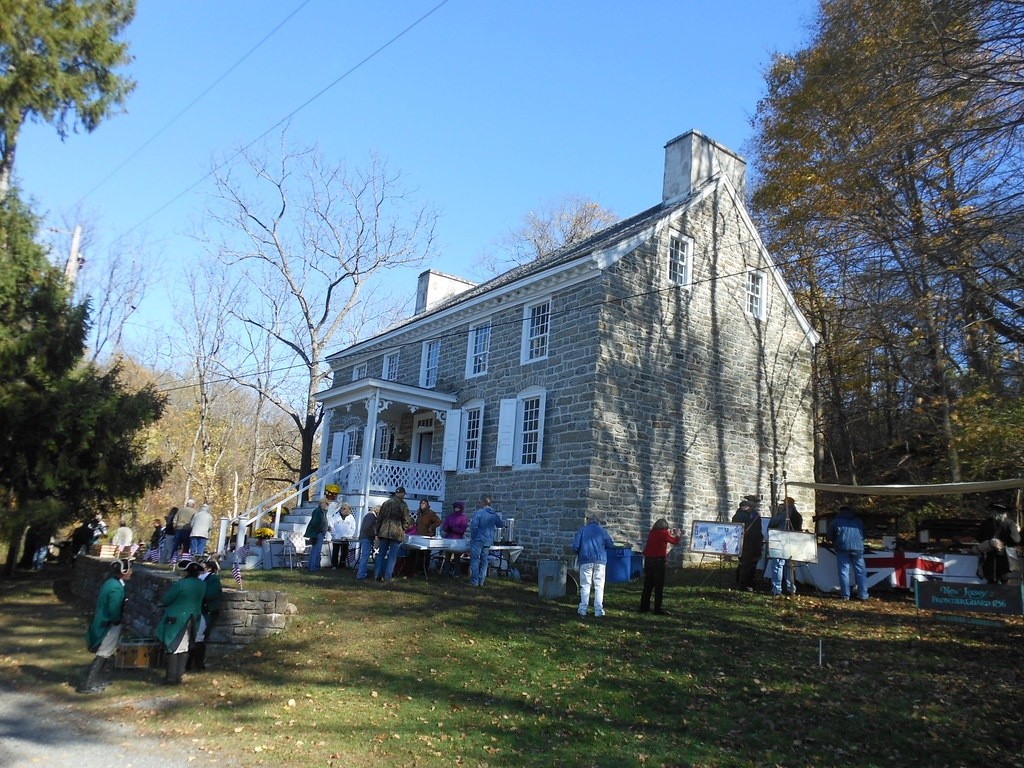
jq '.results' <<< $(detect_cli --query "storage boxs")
[411,537,451,548]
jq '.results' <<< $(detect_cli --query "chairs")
[283,532,312,574]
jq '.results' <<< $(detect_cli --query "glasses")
[455,506,460,509]
[341,507,347,510]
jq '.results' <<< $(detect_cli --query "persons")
[171,500,197,557]
[113,521,132,551]
[388,438,411,494]
[304,486,504,586]
[162,508,178,562]
[20,521,56,569]
[78,557,136,693]
[975,503,1021,585]
[732,495,803,595]
[637,519,682,613]
[152,560,206,685]
[72,519,94,548]
[150,519,161,551]
[185,560,221,674]
[89,515,107,541]
[827,505,869,602]
[572,514,613,618]
[190,505,212,556]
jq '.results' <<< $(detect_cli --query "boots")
[177,653,189,683]
[185,649,194,671]
[97,658,112,686]
[161,654,178,685]
[80,655,105,693]
[194,642,205,672]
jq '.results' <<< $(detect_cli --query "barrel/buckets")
[607,544,643,585]
[537,558,568,603]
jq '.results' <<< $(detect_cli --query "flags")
[239,546,250,557]
[232,564,241,584]
[143,548,193,566]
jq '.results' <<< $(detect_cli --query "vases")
[272,515,275,522]
[256,537,266,545]
[326,492,334,500]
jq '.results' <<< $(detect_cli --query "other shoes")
[640,607,646,612]
[594,611,606,616]
[655,610,665,615]
[578,609,586,616]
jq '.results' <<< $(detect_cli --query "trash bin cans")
[263,538,285,570]
[537,560,568,599]
[606,540,632,582]
[630,551,644,577]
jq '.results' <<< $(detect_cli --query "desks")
[268,540,524,582]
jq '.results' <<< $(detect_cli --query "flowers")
[325,484,340,496]
[268,508,287,515]
[253,527,274,539]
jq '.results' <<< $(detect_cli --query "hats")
[109,557,136,574]
[781,497,794,503]
[744,496,760,502]
[177,559,204,572]
[396,487,407,495]
[740,502,753,507]
[203,556,221,571]
[986,499,1009,511]
[320,498,330,504]
